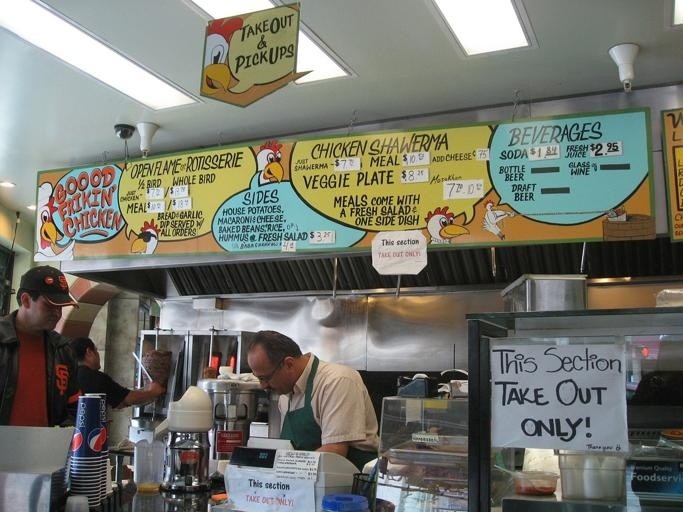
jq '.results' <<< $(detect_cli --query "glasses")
[259,357,285,382]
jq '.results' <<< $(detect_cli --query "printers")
[223,446,362,512]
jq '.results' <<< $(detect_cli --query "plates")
[661,428,683,439]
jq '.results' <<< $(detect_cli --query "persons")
[247,330,382,471]
[0,265,165,428]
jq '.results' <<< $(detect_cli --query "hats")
[20,265,80,312]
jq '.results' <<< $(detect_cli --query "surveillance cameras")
[114,124,135,139]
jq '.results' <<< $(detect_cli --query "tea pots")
[133,439,164,494]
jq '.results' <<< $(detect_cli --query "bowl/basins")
[512,471,559,496]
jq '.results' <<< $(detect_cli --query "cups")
[64,393,113,512]
